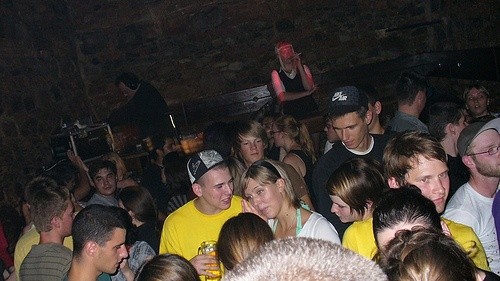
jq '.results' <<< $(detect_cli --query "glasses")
[269,130,280,136]
[159,165,165,170]
[467,145,500,156]
[465,95,488,101]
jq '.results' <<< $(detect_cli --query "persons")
[271,40,318,118]
[0,67,500,281]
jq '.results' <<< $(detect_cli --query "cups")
[201,241,222,281]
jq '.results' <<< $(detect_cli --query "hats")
[263,159,307,201]
[327,86,367,114]
[187,150,230,184]
[457,118,500,158]
[274,40,293,51]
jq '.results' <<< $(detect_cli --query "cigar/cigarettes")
[296,51,302,56]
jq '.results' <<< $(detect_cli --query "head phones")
[130,81,137,89]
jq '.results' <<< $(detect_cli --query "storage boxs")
[51,125,115,164]
